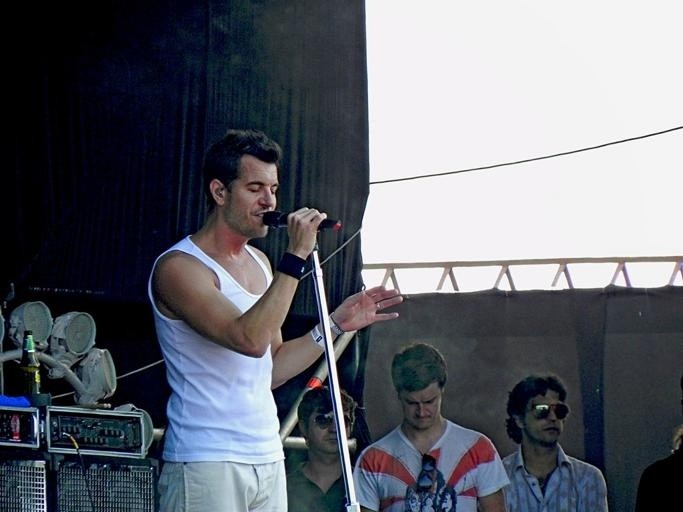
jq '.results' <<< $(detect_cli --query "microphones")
[263,210,343,233]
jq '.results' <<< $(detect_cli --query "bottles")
[20,328,39,396]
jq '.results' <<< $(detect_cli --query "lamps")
[0,302,117,408]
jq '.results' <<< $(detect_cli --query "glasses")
[533,403,569,419]
[416,453,436,493]
[315,414,351,429]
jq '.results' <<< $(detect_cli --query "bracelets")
[310,326,324,347]
[328,312,344,336]
[275,251,306,281]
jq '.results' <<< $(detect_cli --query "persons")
[286,384,363,510]
[633,374,682,511]
[352,340,513,511]
[146,128,405,511]
[497,372,610,511]
